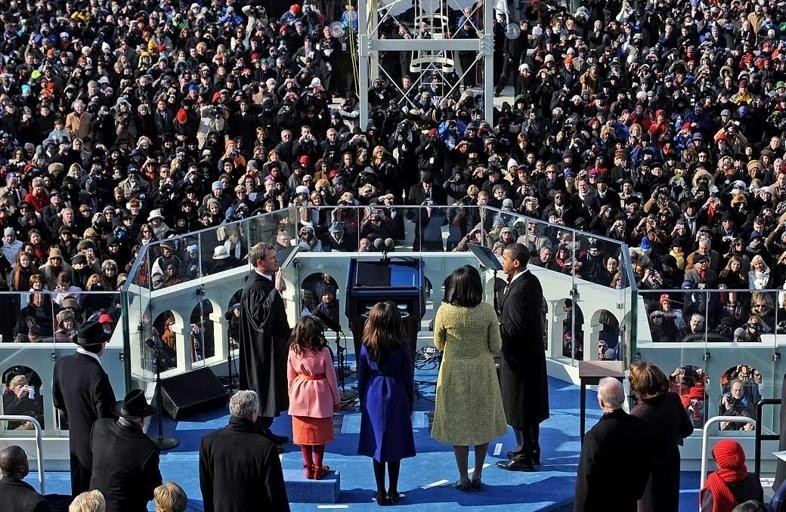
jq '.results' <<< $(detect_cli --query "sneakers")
[266,429,289,453]
[375,493,400,506]
[455,480,482,491]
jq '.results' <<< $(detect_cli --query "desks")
[580,361,625,446]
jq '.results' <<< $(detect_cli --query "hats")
[71,320,111,347]
[110,388,158,420]
[688,385,708,401]
[0,0,786,340]
[713,440,746,470]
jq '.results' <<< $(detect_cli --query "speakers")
[151,366,230,419]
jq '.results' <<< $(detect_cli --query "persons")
[0,0,785,511]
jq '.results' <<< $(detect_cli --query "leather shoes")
[496,451,542,473]
[304,464,330,480]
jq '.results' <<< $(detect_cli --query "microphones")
[384,238,394,251]
[144,338,170,361]
[373,238,384,252]
[312,308,341,333]
